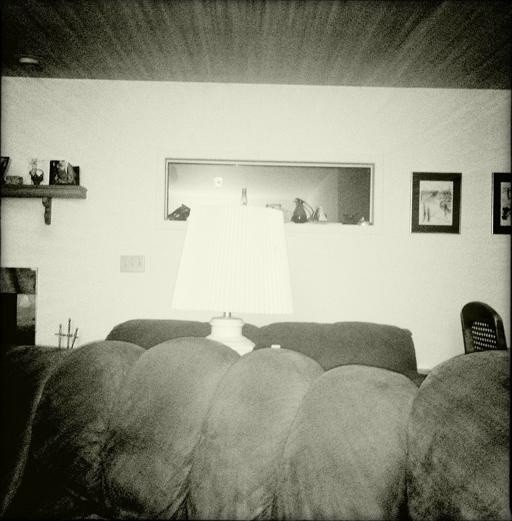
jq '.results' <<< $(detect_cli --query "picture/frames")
[490,170,512,236]
[410,170,462,235]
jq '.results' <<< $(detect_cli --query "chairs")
[460,301,508,354]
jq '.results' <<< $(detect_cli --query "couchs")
[0,340,512,521]
[106,319,428,387]
